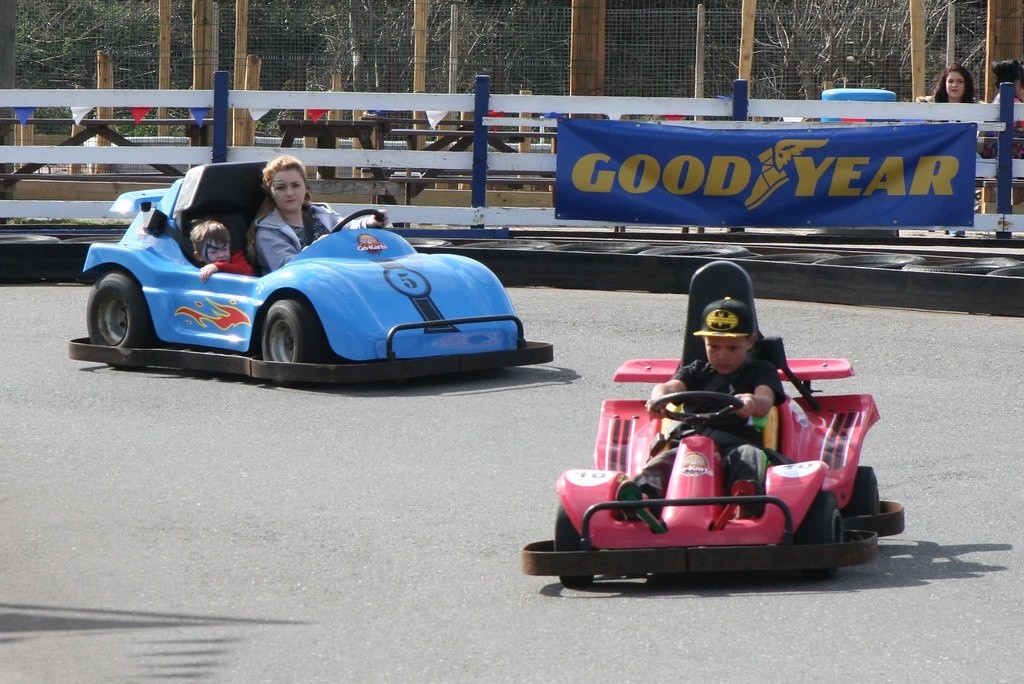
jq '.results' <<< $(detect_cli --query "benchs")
[390,174,556,185]
[0,173,184,183]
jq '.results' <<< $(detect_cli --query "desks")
[0,117,214,185]
[276,119,397,204]
[362,117,522,198]
[393,129,558,198]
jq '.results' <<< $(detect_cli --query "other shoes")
[727,481,764,511]
[615,480,653,520]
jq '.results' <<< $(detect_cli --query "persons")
[916,64,985,237]
[980,60,1024,159]
[247,157,390,273]
[190,221,254,281]
[615,296,787,520]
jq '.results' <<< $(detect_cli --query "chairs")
[681,260,766,367]
[173,160,270,254]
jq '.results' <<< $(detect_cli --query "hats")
[692,296,757,338]
